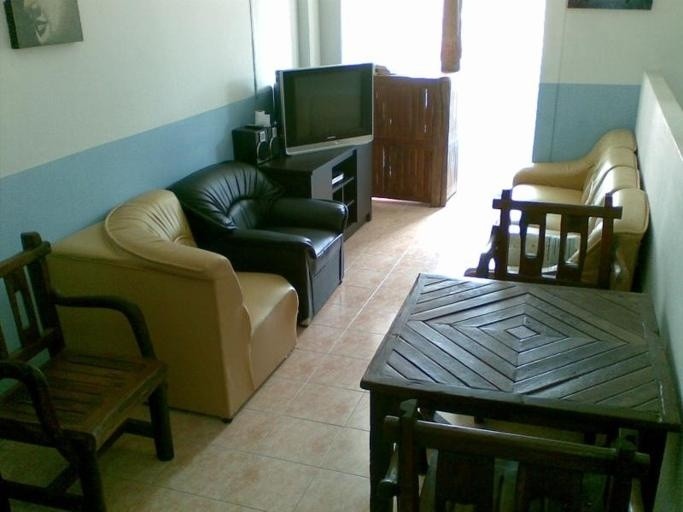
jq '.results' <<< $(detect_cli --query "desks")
[259,141,374,242]
[359,273,683,511]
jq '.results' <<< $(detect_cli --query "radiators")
[245,124,264,130]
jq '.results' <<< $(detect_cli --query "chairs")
[1,232,173,512]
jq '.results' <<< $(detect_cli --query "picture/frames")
[259,141,374,242]
[359,273,683,511]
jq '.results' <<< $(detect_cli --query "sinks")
[463,128,650,288]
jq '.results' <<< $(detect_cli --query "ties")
[274,62,375,155]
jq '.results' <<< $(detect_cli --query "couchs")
[45,189,299,422]
[463,128,650,288]
[169,161,348,326]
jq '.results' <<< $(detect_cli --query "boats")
[267,125,283,160]
[232,127,270,165]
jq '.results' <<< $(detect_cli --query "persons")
[22,0,78,45]
